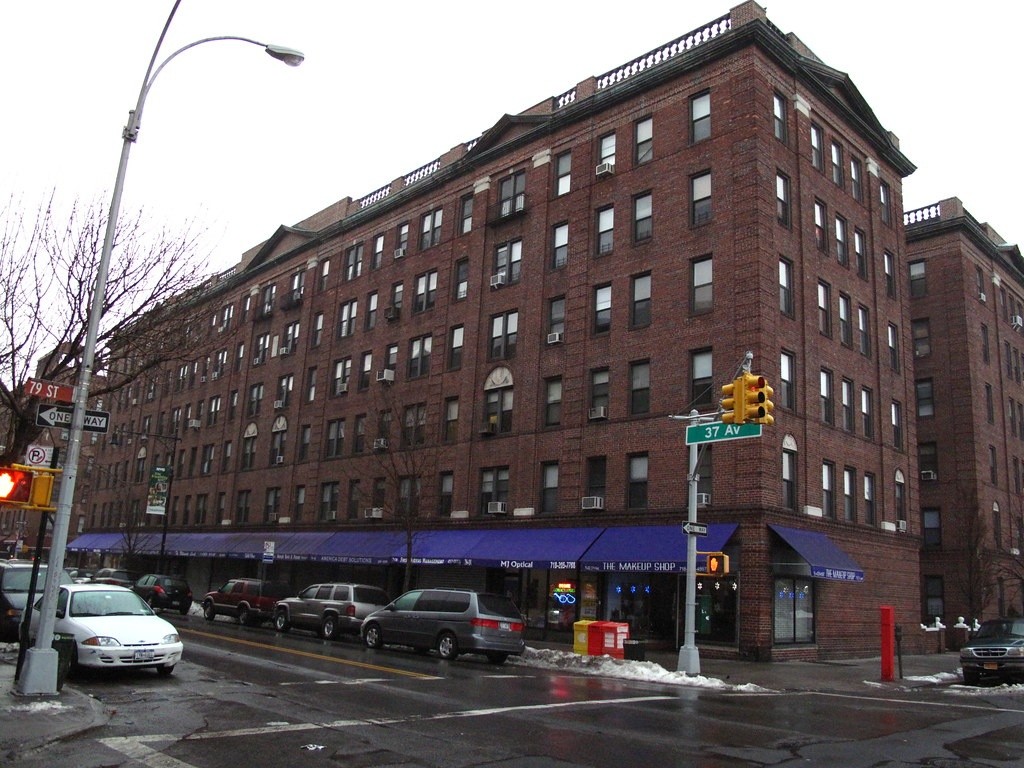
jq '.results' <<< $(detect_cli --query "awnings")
[47,521,742,575]
[768,520,869,583]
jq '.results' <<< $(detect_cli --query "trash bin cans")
[623,638,645,662]
[31,631,75,691]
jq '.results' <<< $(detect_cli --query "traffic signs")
[685,419,762,446]
[36,404,109,435]
[24,377,74,403]
[681,520,708,537]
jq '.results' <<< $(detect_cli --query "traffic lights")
[0,467,34,505]
[706,553,730,575]
[743,372,765,420]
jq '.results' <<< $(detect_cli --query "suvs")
[128,574,193,616]
[0,558,74,640]
[960,615,1023,687]
[92,569,138,592]
[272,581,394,639]
[200,576,284,627]
[360,584,526,666]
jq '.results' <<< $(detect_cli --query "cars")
[19,580,183,685]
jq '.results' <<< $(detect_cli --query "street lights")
[895,622,904,679]
[109,426,180,574]
[10,2,306,696]
